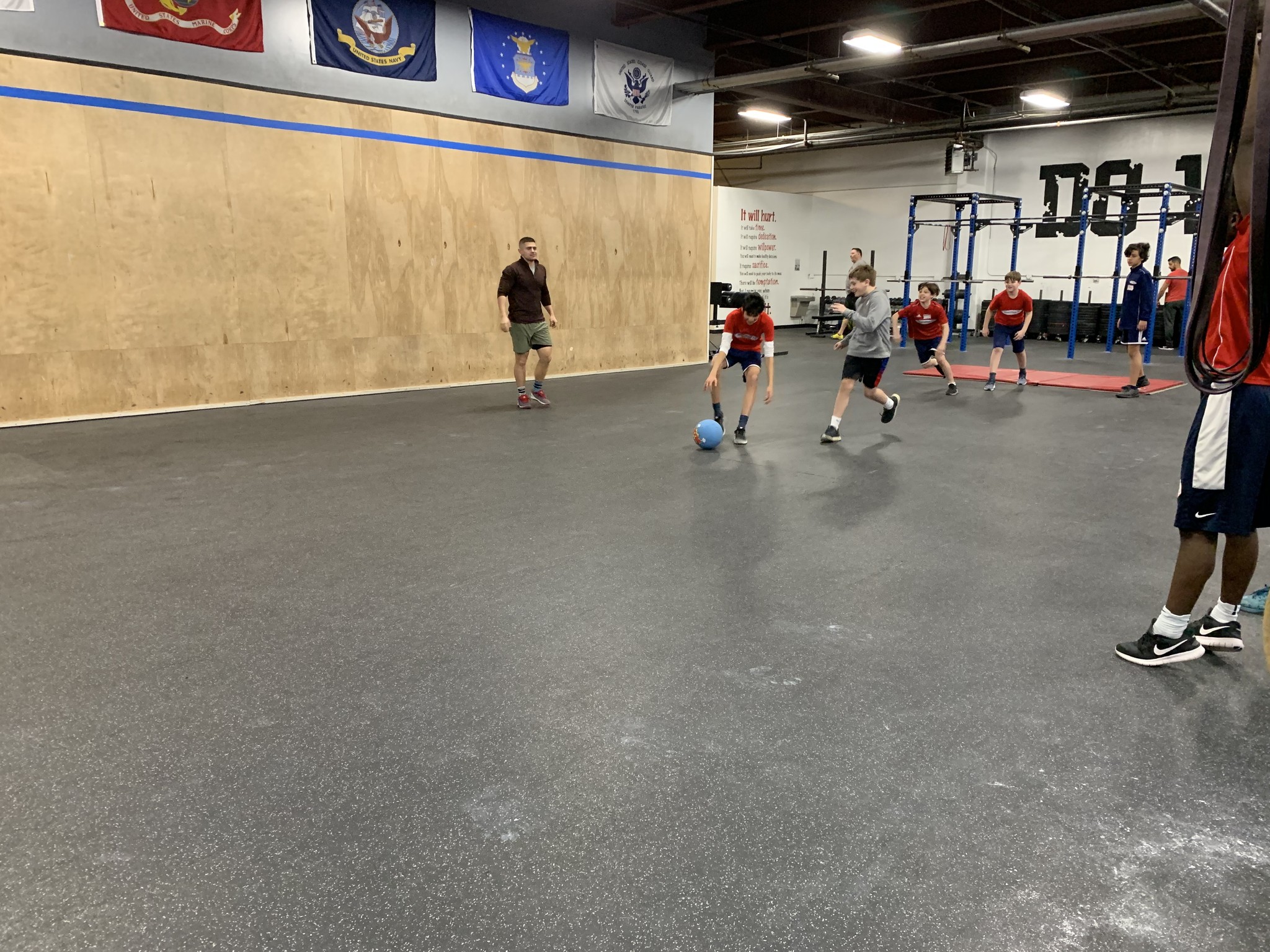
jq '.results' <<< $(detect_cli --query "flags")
[96,0,265,53]
[594,38,673,126]
[306,0,437,82]
[468,7,569,106]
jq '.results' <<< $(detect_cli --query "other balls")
[693,418,723,451]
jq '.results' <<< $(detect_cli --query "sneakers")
[984,375,995,391]
[1240,584,1270,613]
[517,393,532,409]
[1115,386,1140,398]
[934,363,944,375]
[1016,369,1027,385]
[1186,607,1244,652]
[945,384,958,396]
[879,394,900,424]
[1158,345,1174,350]
[733,426,747,444]
[831,333,844,340]
[1135,377,1150,388]
[820,426,841,442]
[713,412,726,436]
[1114,618,1205,666]
[531,390,550,405]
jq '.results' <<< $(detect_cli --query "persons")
[981,271,1033,391]
[1115,242,1153,397]
[820,264,900,441]
[1115,36,1269,668]
[497,236,557,408]
[703,292,774,444]
[1156,256,1188,349]
[893,281,959,396]
[832,247,866,342]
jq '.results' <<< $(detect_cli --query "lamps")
[1020,89,1071,109]
[737,106,792,123]
[842,28,901,54]
